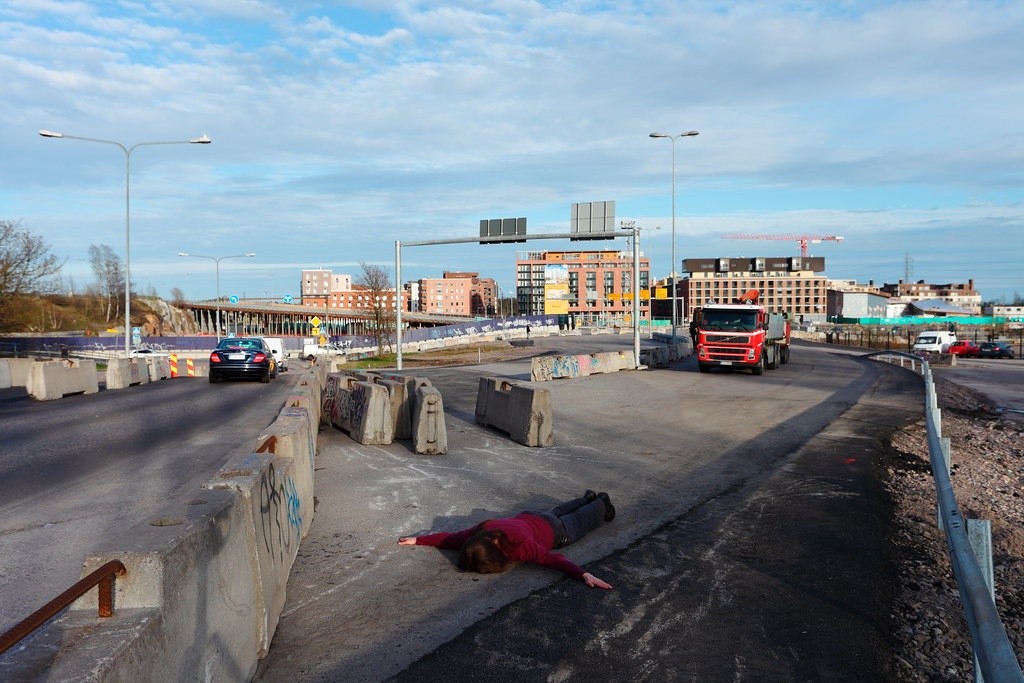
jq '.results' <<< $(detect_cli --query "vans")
[263,338,290,372]
[913,331,957,354]
[303,344,346,362]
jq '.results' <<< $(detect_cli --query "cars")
[978,342,1015,359]
[209,337,277,383]
[948,340,980,358]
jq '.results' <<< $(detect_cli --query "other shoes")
[584,490,597,502]
[597,492,615,522]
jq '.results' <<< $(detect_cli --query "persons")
[397,488,616,590]
[708,295,716,304]
[684,316,689,324]
[527,322,532,339]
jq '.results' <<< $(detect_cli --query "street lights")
[650,130,699,364]
[178,253,256,344]
[38,130,212,359]
[637,226,661,339]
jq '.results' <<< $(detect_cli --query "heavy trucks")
[689,290,793,376]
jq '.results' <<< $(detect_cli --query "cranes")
[720,234,844,257]
